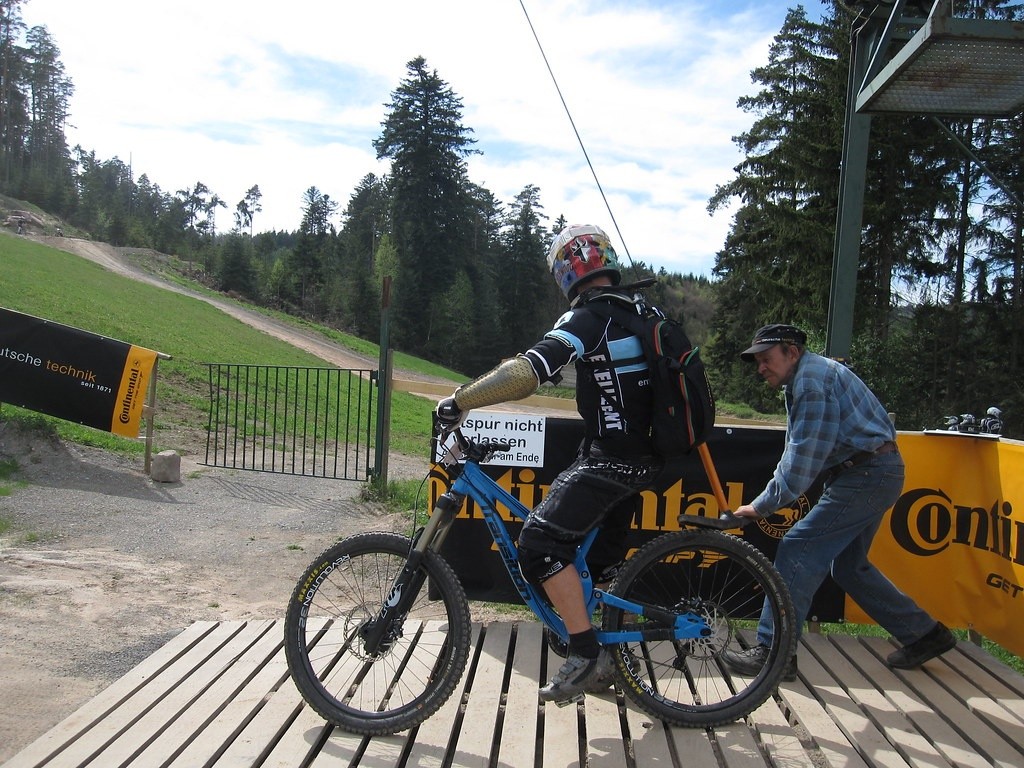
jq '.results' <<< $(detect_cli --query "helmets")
[546,224,622,302]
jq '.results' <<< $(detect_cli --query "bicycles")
[283,399,798,739]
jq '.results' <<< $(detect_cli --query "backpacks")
[582,302,715,454]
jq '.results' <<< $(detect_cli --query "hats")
[740,324,807,363]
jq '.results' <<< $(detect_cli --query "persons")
[17,219,24,233]
[435,224,664,702]
[733,324,957,681]
[944,416,959,431]
[960,414,975,431]
[981,407,1003,433]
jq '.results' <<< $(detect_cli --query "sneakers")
[887,621,956,669]
[721,644,797,681]
[538,646,610,702]
[584,648,641,693]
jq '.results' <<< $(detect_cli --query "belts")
[831,441,898,474]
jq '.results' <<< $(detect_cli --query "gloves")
[434,388,469,446]
